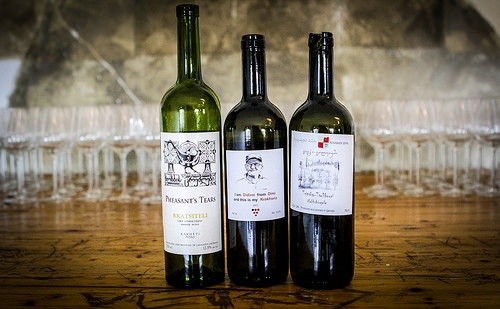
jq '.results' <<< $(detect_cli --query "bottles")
[289,31,354,289]
[224,34,288,289]
[160,4,225,288]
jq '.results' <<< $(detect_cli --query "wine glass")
[0,89,500,205]
